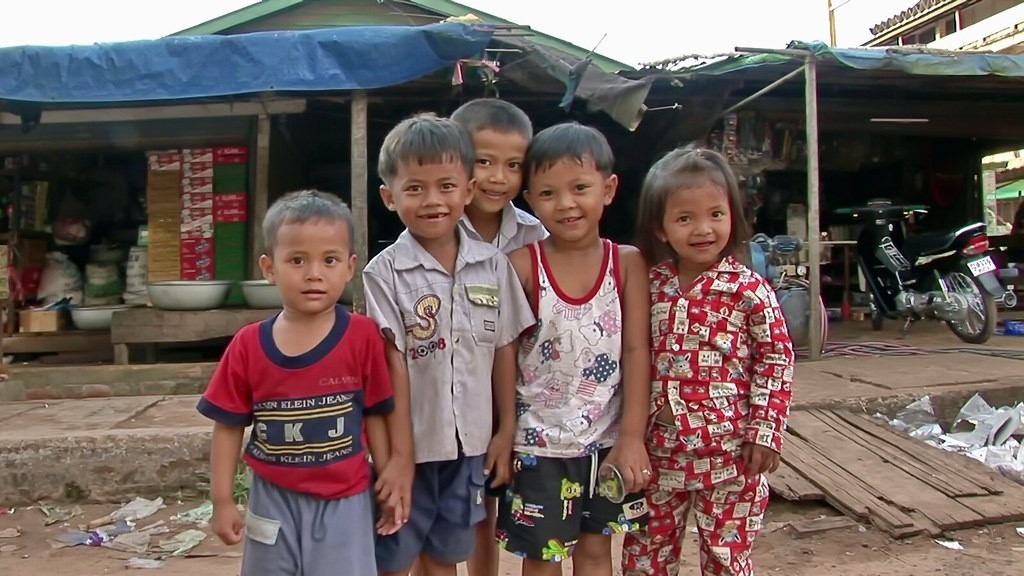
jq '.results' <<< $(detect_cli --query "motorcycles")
[834,197,1017,344]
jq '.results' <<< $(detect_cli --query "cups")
[598,463,629,503]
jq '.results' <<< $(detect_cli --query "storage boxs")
[18,310,71,332]
[84,146,246,306]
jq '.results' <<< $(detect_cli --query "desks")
[109,307,283,366]
[801,240,857,302]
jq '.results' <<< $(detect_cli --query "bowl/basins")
[142,280,235,311]
[68,304,146,330]
[1003,320,1024,336]
[239,279,283,309]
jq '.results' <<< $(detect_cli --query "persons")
[410,98,550,576]
[1009,201,1023,256]
[622,145,795,576]
[494,122,653,576]
[362,112,536,576]
[197,189,403,576]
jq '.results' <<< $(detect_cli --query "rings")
[642,470,652,476]
